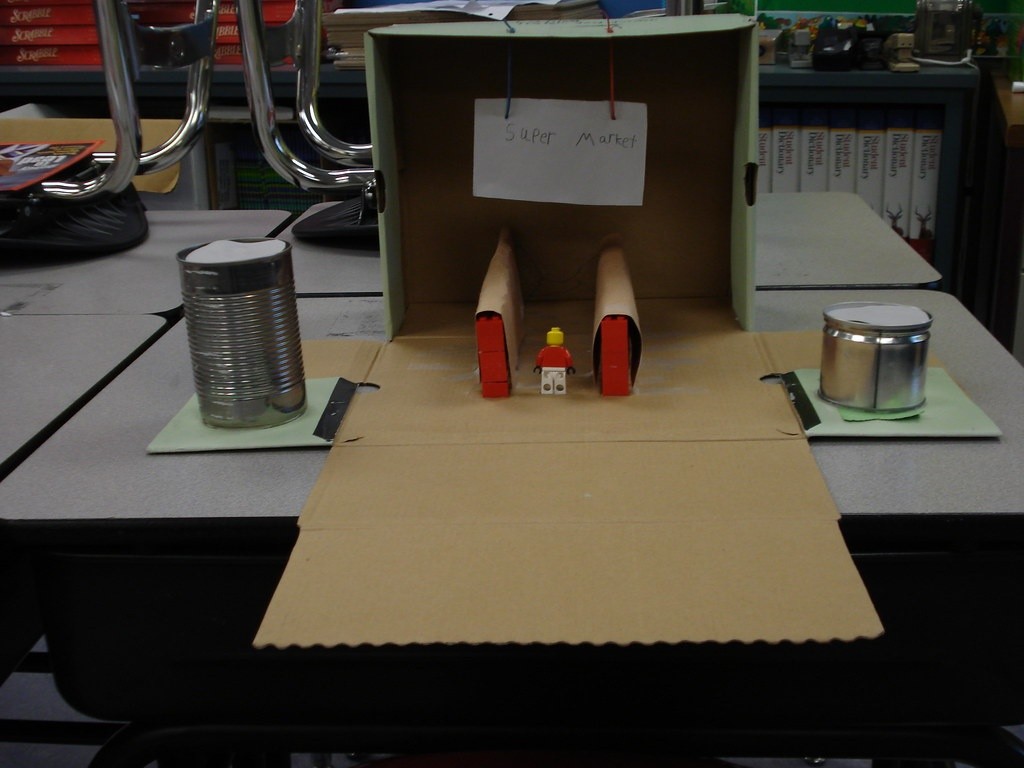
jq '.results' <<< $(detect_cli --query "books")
[0,138,105,191]
[322,0,604,70]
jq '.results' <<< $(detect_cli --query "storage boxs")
[147,12,1004,653]
[138,132,236,209]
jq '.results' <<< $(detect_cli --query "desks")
[0,191,1024,768]
[0,57,987,319]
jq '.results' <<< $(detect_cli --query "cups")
[176,237,309,430]
[815,302,934,414]
